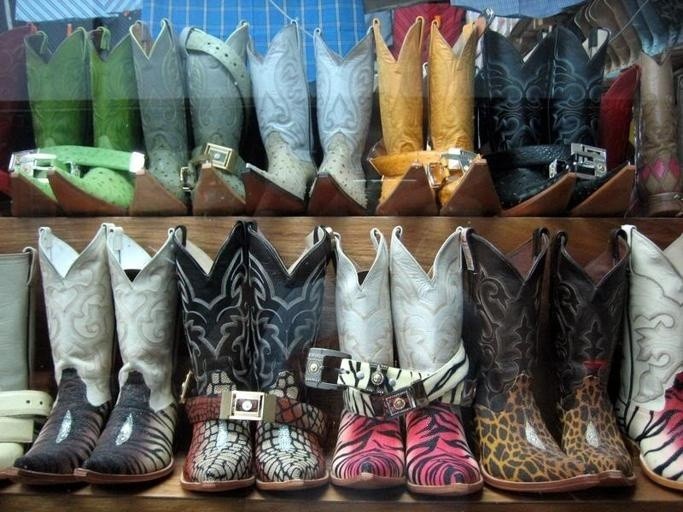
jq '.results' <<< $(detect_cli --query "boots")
[0,221,683,493]
[9,17,683,215]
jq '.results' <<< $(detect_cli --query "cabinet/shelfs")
[0,210,683,512]
